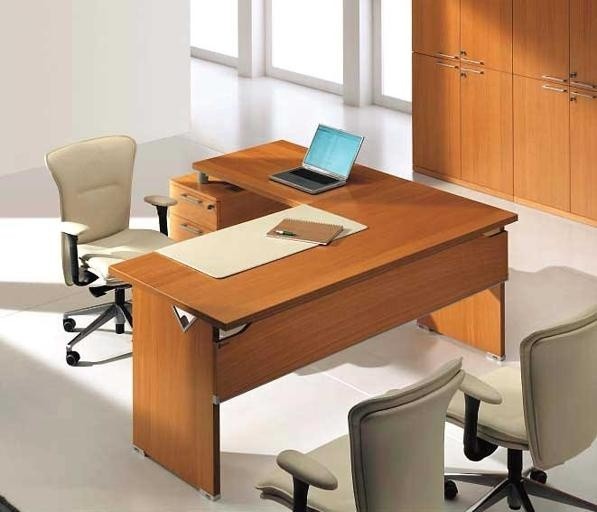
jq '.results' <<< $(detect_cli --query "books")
[265,217,342,246]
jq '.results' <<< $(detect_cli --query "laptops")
[269,123,365,195]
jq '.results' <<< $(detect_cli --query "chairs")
[379,302,597,511]
[250,354,464,510]
[44,134,178,366]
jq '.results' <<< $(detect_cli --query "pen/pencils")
[273,230,296,236]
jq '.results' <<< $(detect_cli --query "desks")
[107,139,517,504]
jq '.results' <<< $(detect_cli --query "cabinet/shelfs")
[509,69,597,229]
[507,0,597,95]
[411,0,512,75]
[410,53,513,206]
